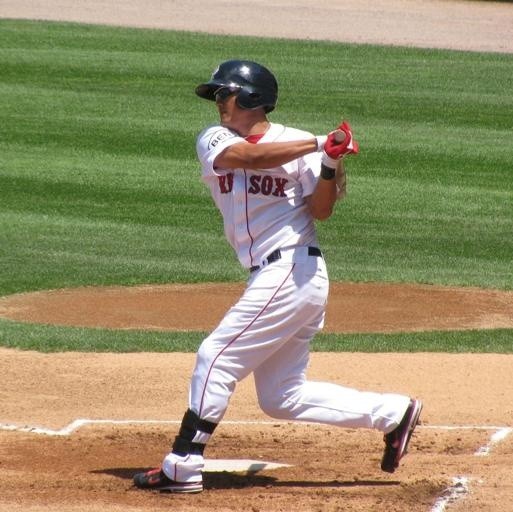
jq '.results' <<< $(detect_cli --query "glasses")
[213,87,238,101]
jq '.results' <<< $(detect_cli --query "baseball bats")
[334,128,346,200]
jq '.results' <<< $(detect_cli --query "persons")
[135,59,424,494]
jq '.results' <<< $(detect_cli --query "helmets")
[195,60,278,113]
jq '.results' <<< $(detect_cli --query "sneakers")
[380,397,424,474]
[132,467,203,494]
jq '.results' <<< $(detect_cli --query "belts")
[249,246,322,272]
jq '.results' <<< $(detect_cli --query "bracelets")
[320,163,335,180]
[317,136,327,152]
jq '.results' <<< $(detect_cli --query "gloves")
[315,136,359,155]
[323,121,353,169]
